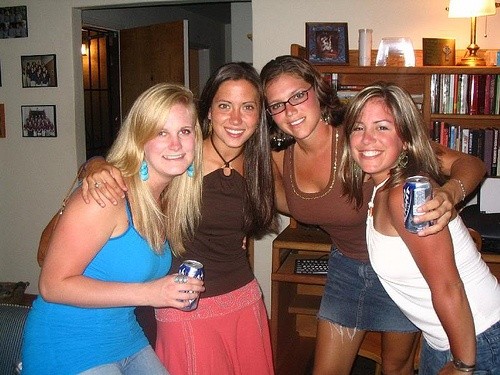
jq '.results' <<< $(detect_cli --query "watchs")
[453,358,476,368]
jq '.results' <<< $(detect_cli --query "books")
[429,120,500,177]
[429,73,500,115]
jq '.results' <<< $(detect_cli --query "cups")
[359,29,372,67]
[422,37,457,66]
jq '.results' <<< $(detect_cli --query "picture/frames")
[21,54,58,88]
[21,105,58,138]
[0,5,29,39]
[305,22,349,66]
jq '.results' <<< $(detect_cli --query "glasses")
[265,84,313,116]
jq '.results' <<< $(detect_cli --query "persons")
[259,55,488,375]
[20,82,206,375]
[23,118,54,136]
[37,61,291,375]
[25,62,52,86]
[338,81,500,375]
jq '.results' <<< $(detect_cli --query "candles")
[358,29,372,67]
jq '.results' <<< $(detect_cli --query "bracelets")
[452,362,476,372]
[448,179,466,201]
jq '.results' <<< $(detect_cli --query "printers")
[461,174,500,254]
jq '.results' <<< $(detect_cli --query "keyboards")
[293,258,330,275]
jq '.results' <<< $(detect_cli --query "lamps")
[448,0,496,66]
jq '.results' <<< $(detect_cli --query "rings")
[173,275,188,284]
[95,181,103,188]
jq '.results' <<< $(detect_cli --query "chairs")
[0,281,158,375]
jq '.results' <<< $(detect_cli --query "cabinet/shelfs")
[271,45,500,375]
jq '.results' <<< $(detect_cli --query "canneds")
[402,176,434,233]
[178,260,204,311]
